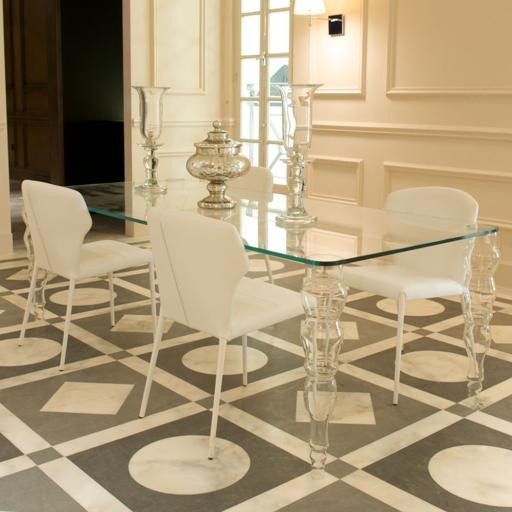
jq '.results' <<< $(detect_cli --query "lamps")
[294,0,344,36]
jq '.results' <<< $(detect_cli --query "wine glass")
[131,86,172,192]
[136,190,166,219]
[275,222,316,258]
[272,83,324,225]
